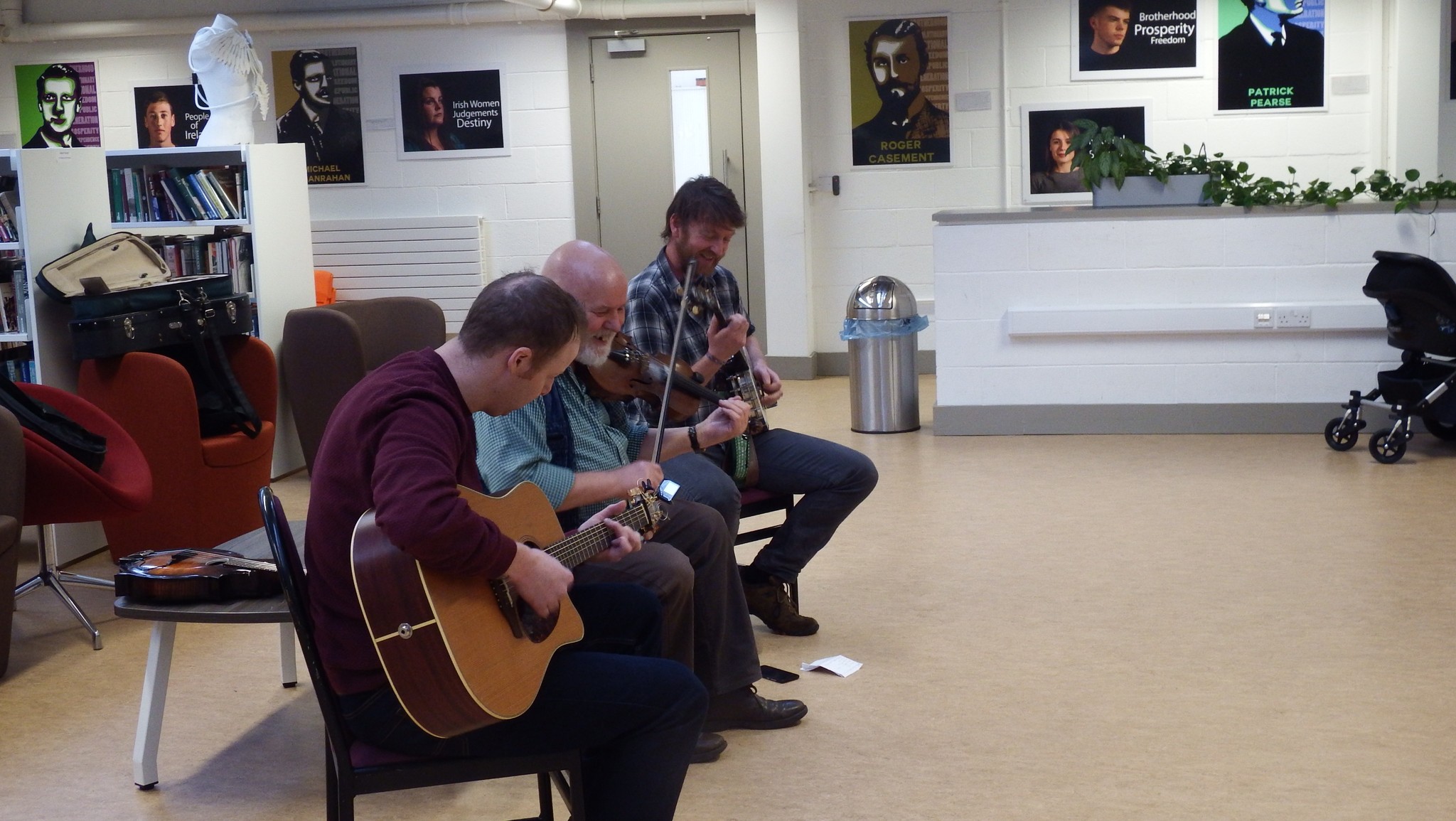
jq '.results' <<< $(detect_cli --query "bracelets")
[705,352,727,365]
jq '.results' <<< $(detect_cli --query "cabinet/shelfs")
[0,147,112,572]
[105,143,317,477]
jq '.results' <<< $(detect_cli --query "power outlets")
[1277,308,1310,327]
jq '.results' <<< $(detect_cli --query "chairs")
[733,485,798,614]
[258,485,583,821]
[14,381,151,649]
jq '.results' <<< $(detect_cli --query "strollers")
[1326,249,1456,467]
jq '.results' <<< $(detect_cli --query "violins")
[588,331,758,441]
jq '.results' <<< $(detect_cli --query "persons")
[1030,120,1091,195]
[616,176,879,637]
[851,19,950,167]
[471,240,807,763]
[1079,1,1134,70]
[188,14,269,147]
[304,268,697,821]
[21,64,84,149]
[276,50,364,183]
[138,91,179,147]
[402,77,464,152]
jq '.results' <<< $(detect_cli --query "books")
[0,173,36,384]
[111,165,248,223]
[135,224,260,338]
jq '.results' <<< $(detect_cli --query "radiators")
[310,215,488,334]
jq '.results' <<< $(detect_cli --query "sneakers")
[740,566,819,636]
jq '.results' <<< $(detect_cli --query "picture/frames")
[1214,0,1329,115]
[270,42,368,187]
[11,59,104,149]
[844,12,954,169]
[129,79,211,149]
[1021,98,1150,204]
[1071,0,1202,82]
[392,62,512,160]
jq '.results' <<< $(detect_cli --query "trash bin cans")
[844,275,920,434]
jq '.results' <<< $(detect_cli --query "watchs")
[687,424,708,453]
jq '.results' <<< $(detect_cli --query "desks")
[113,519,306,792]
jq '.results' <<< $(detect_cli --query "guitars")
[351,478,665,740]
[114,550,285,606]
[670,281,769,433]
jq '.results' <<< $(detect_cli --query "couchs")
[282,296,446,478]
[80,336,279,565]
[0,405,24,675]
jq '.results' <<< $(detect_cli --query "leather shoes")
[685,730,727,763]
[706,684,809,731]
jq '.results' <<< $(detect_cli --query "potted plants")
[1065,120,1456,238]
